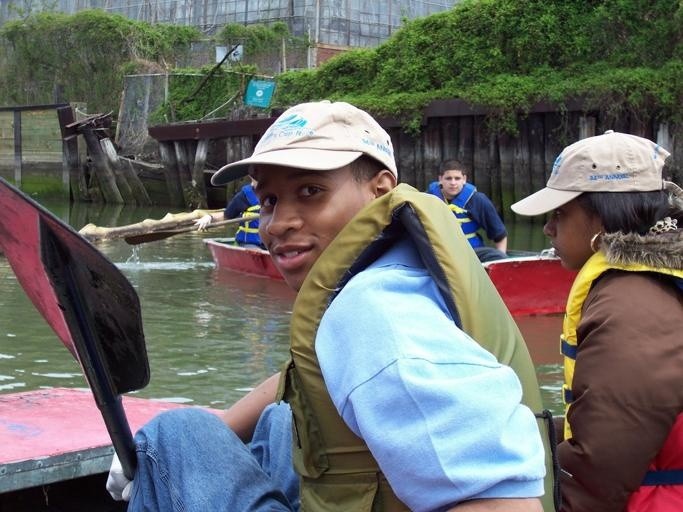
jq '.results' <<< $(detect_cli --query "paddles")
[0,179,150,483]
[124,215,260,246]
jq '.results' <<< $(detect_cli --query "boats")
[0,388,568,512]
[201,236,580,318]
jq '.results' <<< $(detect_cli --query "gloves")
[105,451,135,502]
[192,215,212,231]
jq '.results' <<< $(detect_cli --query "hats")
[211,100,398,187]
[510,130,683,216]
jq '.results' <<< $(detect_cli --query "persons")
[105,101,557,512]
[426,160,508,263]
[510,129,683,512]
[191,174,267,252]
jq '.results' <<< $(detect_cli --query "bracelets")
[209,214,213,221]
